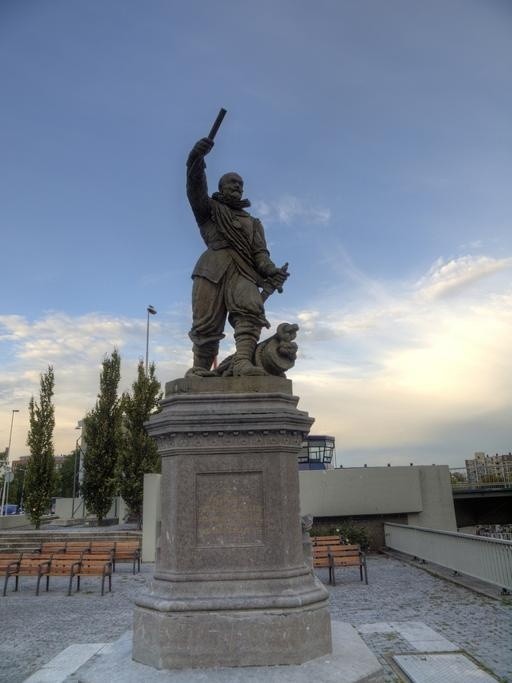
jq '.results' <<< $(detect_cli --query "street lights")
[7,410,20,465]
[146,305,156,377]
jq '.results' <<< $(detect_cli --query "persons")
[184,136,289,376]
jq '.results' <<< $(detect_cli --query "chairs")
[310,534,369,587]
[0,530,140,597]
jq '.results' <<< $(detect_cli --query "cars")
[2,505,20,515]
